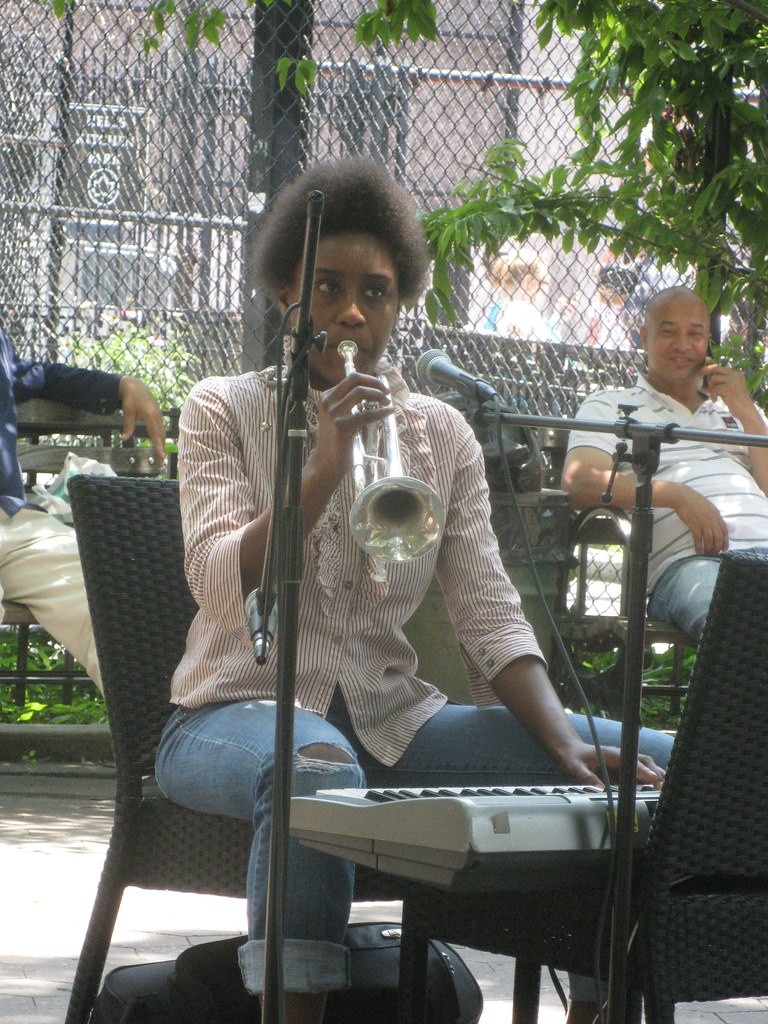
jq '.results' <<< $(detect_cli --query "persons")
[479,239,669,355]
[560,283,768,643]
[153,157,677,1023]
[0,315,166,699]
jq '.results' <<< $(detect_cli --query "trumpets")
[333,338,447,563]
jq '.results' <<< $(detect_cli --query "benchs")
[0,398,181,767]
[538,442,697,723]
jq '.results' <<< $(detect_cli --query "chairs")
[64,475,542,1024]
[394,547,768,1024]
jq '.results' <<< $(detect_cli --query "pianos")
[288,779,666,899]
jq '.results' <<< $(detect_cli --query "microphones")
[245,588,279,665]
[416,348,498,400]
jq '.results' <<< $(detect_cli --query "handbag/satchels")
[89,921,484,1024]
